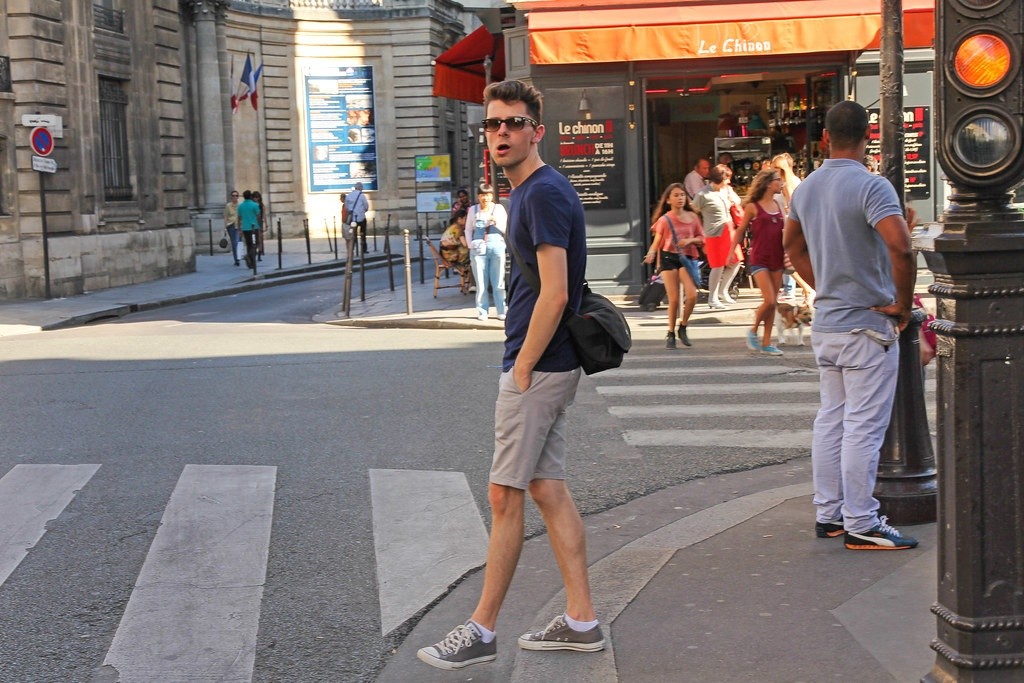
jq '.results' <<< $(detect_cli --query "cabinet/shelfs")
[714,136,771,197]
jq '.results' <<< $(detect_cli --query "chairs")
[426,238,467,298]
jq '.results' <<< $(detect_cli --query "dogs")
[775,302,812,346]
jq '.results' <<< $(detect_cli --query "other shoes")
[759,345,784,356]
[478,315,488,321]
[746,328,759,350]
[469,285,476,292]
[497,313,506,320]
[364,250,370,253]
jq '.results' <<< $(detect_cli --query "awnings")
[524,0,936,64]
[431,24,506,104]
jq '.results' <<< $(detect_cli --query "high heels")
[718,291,736,304]
[708,298,726,309]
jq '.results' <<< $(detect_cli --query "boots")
[258,251,262,261]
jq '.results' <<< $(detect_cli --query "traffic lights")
[936,1,1024,190]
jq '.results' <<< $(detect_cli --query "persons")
[416,80,607,670]
[339,182,369,256]
[782,99,919,550]
[223,189,267,269]
[438,182,507,321]
[643,152,935,366]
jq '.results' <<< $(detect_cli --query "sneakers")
[843,515,919,550]
[665,330,677,350]
[677,322,692,346]
[517,612,605,653]
[416,620,497,671]
[815,516,845,538]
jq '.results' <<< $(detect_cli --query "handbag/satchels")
[347,216,352,225]
[470,238,486,256]
[569,278,632,375]
[220,238,228,248]
[703,221,744,269]
[236,235,248,260]
[680,255,701,286]
[730,204,745,227]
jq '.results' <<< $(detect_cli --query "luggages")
[638,255,709,312]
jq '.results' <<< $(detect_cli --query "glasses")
[481,115,539,132]
[769,177,783,182]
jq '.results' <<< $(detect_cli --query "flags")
[229,51,262,115]
